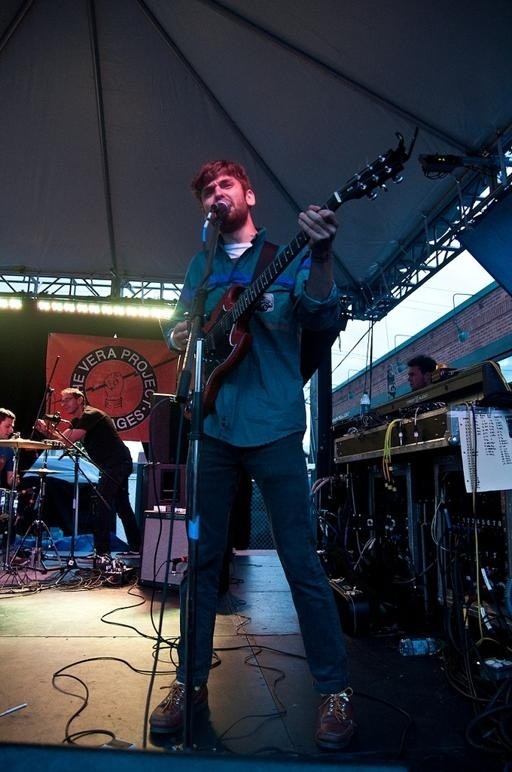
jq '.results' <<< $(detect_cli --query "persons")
[0,407,32,568]
[33,387,142,560]
[406,352,437,390]
[148,158,360,751]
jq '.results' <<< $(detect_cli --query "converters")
[102,737,136,752]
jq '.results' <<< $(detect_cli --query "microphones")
[40,413,60,424]
[210,199,232,213]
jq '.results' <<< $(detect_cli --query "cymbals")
[23,468,63,473]
[0,438,53,449]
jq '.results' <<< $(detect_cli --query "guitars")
[176,127,419,421]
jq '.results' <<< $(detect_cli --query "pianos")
[333,360,512,464]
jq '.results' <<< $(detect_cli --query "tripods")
[9,479,63,573]
[55,457,96,586]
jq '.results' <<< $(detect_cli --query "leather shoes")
[316,688,356,749]
[149,680,208,733]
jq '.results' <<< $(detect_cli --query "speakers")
[136,510,190,591]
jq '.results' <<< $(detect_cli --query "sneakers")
[78,554,94,564]
[116,550,141,559]
[4,554,30,566]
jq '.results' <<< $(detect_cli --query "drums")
[0,488,18,518]
